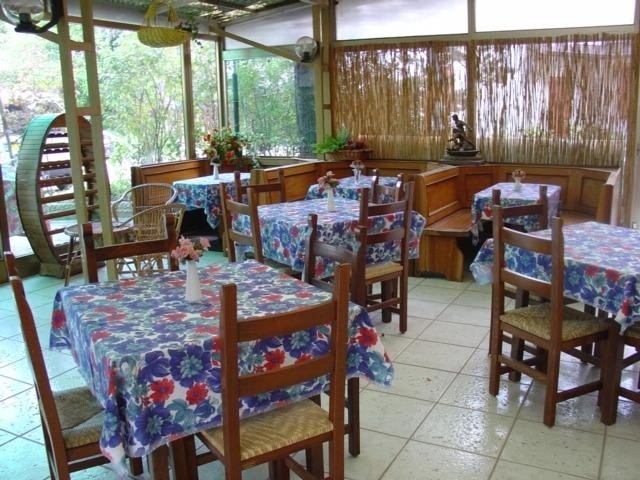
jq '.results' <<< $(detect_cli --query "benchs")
[61,168,624,286]
[137,159,212,209]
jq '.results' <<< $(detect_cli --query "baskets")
[136,0,189,48]
[329,150,372,161]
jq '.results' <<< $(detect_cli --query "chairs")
[596,315,639,426]
[328,182,413,333]
[366,168,409,317]
[296,209,369,457]
[491,183,596,385]
[172,262,354,480]
[4,249,142,479]
[484,203,612,426]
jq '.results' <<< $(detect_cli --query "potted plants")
[310,127,374,162]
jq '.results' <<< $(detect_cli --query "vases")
[220,160,251,173]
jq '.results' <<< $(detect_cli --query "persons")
[447,114,475,150]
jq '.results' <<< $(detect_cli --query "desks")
[50,258,395,480]
[466,220,640,425]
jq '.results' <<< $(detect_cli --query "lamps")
[294,36,318,63]
[0,0,65,34]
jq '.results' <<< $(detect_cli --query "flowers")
[202,127,257,166]
